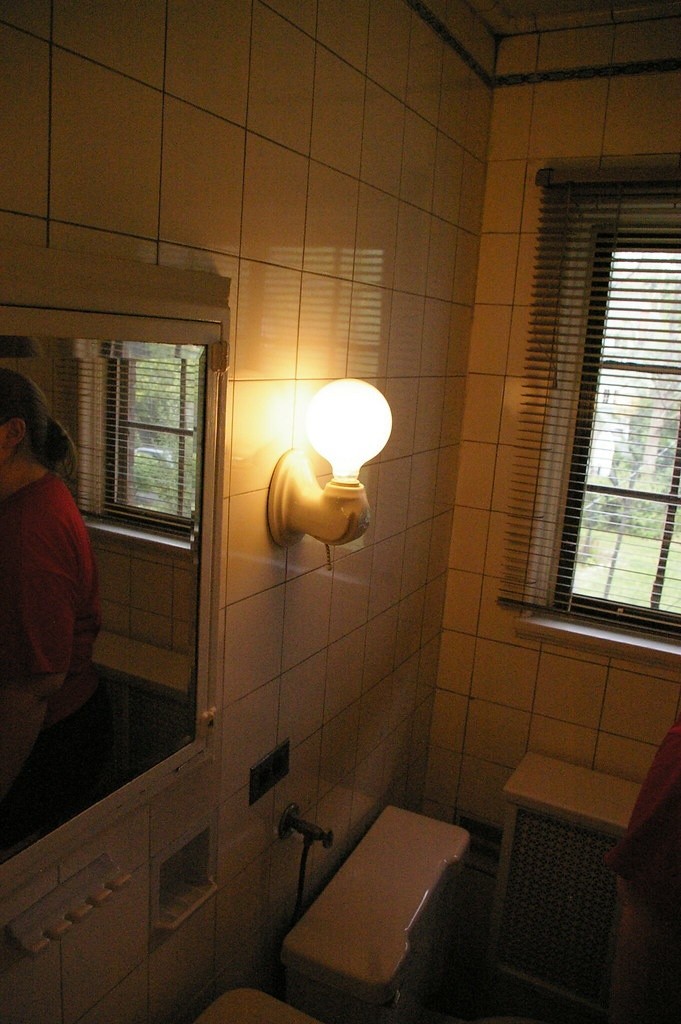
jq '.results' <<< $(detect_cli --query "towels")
[605,716,680,949]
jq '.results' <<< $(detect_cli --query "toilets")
[278,802,470,1023]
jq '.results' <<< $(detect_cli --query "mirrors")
[0,243,231,922]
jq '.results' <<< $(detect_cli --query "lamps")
[265,377,394,556]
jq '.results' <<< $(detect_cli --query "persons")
[0,366,111,853]
[606,710,681,1024]
